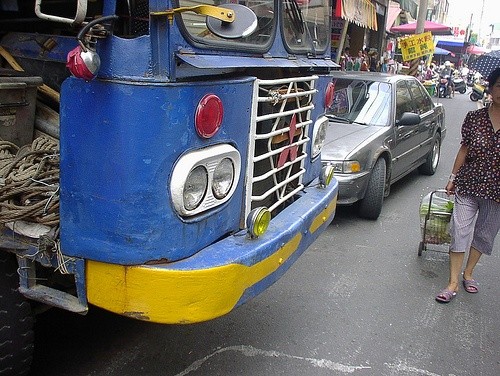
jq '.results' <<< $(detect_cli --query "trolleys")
[418,186,455,256]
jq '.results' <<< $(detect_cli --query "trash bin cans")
[423,84,436,96]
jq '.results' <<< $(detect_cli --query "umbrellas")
[433,47,456,57]
[466,50,500,79]
[390,20,454,35]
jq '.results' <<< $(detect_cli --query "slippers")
[435,288,456,302]
[463,280,479,292]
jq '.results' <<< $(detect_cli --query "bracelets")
[448,173,456,182]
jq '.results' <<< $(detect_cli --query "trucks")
[0,0,341,376]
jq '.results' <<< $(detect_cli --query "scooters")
[466,72,475,86]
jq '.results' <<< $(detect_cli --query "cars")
[321,71,446,220]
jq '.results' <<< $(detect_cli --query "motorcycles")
[468,80,489,102]
[451,73,467,94]
[438,75,449,98]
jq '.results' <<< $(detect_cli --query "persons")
[339,47,389,72]
[387,55,455,98]
[461,64,483,85]
[435,67,500,301]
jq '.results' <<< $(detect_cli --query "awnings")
[333,0,378,31]
